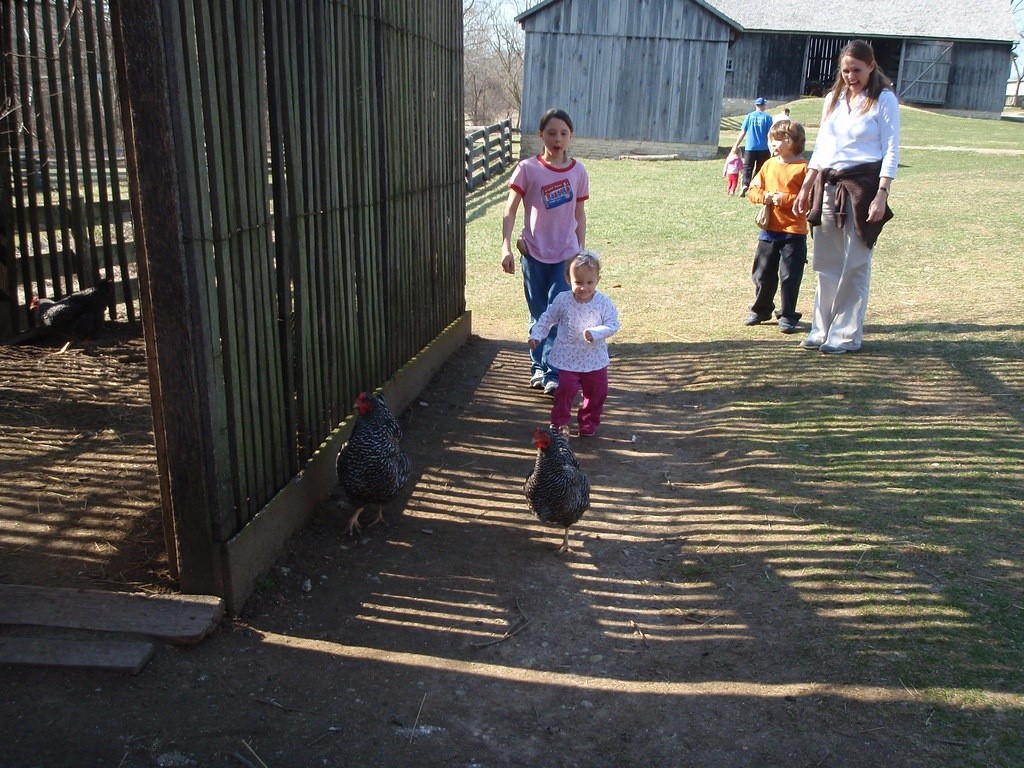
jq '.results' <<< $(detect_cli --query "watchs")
[877,186,890,197]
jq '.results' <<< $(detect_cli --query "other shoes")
[740,186,749,198]
[578,423,596,436]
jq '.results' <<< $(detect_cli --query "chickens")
[523,424,590,556]
[335,386,412,538]
[30,280,107,357]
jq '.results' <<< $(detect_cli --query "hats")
[755,97,768,105]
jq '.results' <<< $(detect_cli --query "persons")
[723,147,745,195]
[743,120,812,333]
[792,39,901,354]
[527,253,620,436]
[772,108,791,124]
[501,108,589,392]
[732,97,774,199]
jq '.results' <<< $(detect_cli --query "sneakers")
[543,381,559,396]
[743,309,772,326]
[530,369,545,389]
[779,321,795,333]
[818,344,846,353]
[801,340,821,349]
